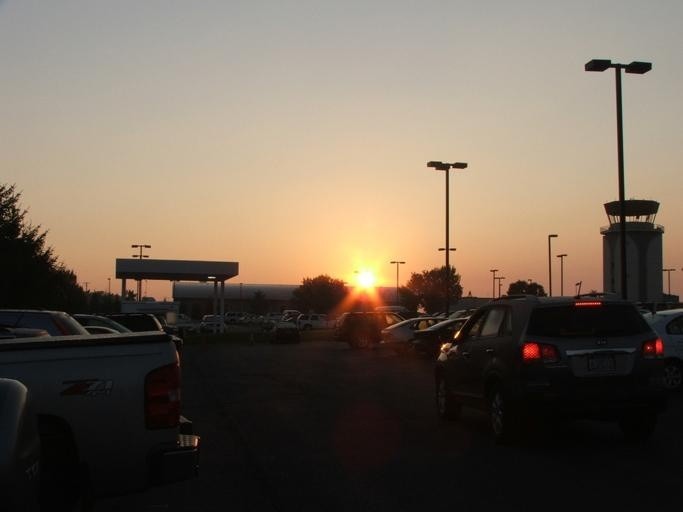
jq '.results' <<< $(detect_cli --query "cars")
[199,314,227,336]
[225,304,480,360]
[2,308,204,512]
[642,307,682,392]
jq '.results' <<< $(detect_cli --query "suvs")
[434,298,671,444]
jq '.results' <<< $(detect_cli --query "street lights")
[106,242,180,304]
[661,265,677,294]
[390,260,405,304]
[487,231,580,298]
[426,156,468,317]
[353,269,369,311]
[582,55,654,302]
[437,246,456,299]
